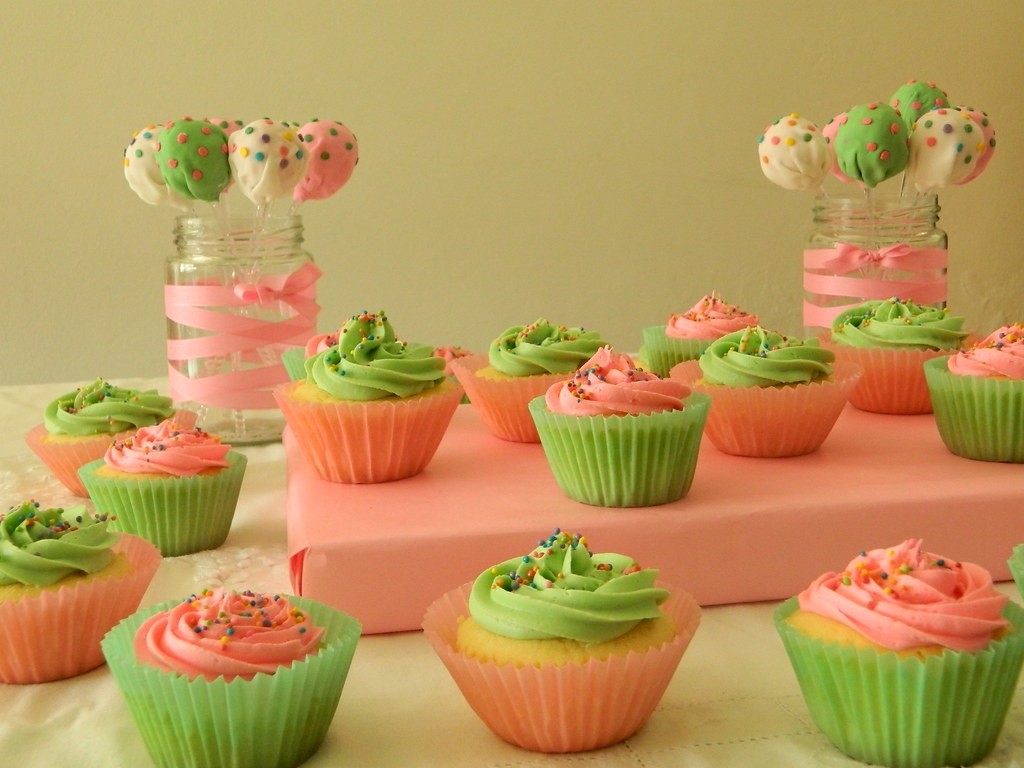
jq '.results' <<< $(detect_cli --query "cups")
[163,214,318,443]
[803,194,949,365]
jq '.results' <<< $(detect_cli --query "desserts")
[1,78,1024,768]
